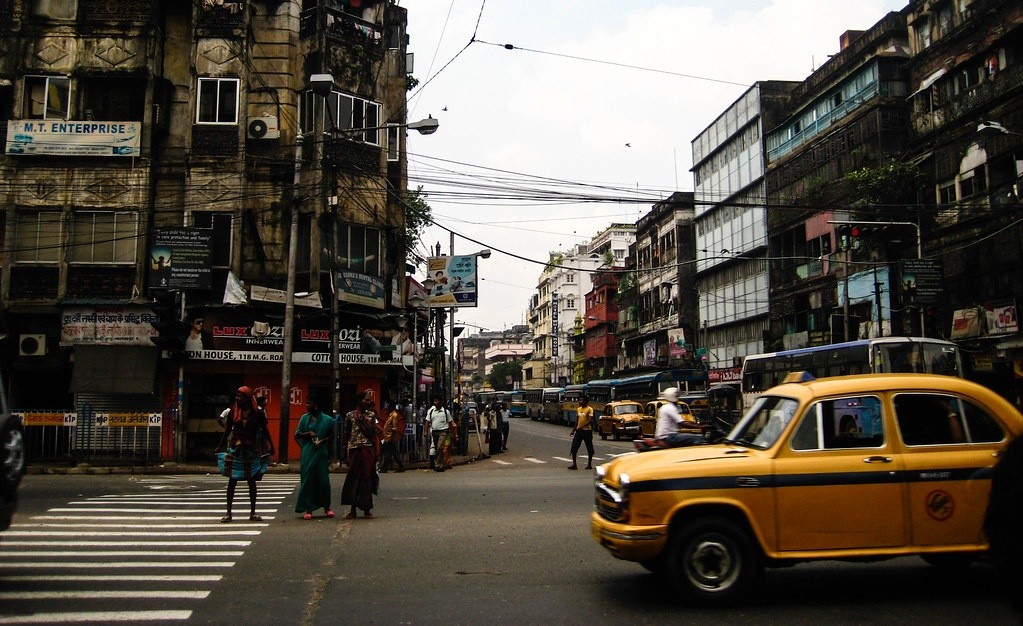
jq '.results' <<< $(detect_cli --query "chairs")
[830,414,858,450]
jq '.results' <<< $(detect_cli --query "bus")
[741,337,963,422]
[474,370,712,435]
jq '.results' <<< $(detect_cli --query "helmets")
[663,387,680,402]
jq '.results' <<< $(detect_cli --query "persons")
[333,390,385,519]
[421,396,459,473]
[364,319,412,361]
[182,314,216,351]
[487,402,512,455]
[378,403,407,473]
[435,271,448,285]
[567,395,595,470]
[293,391,337,520]
[217,384,275,522]
[940,399,962,442]
[653,386,704,446]
[669,334,682,348]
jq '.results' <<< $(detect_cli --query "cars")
[597,400,701,441]
[456,401,480,431]
[591,372,1023,605]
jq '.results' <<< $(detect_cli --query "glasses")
[193,320,205,324]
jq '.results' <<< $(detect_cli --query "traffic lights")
[836,224,896,238]
[926,308,937,337]
[149,290,175,347]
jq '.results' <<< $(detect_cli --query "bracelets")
[948,412,957,418]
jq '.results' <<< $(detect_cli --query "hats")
[232,385,259,424]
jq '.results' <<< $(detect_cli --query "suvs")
[0,378,29,531]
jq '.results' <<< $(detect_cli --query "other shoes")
[345,511,357,519]
[220,514,233,522]
[491,450,505,456]
[250,514,264,521]
[395,467,407,472]
[378,469,388,474]
[324,510,334,516]
[568,465,578,470]
[434,467,445,472]
[502,447,507,450]
[364,511,374,519]
[584,465,592,470]
[303,513,312,519]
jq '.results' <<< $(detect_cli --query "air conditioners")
[19,334,47,355]
[247,116,279,139]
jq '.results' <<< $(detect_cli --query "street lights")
[589,316,616,367]
[511,350,517,389]
[278,118,440,463]
[449,232,492,420]
